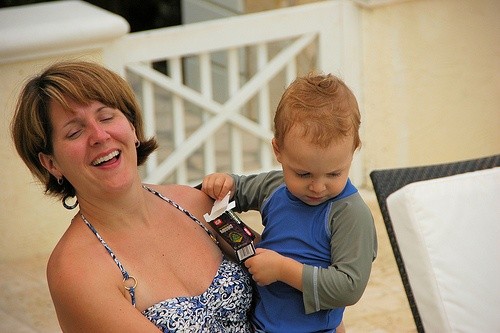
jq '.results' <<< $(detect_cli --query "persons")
[11,61,345,333]
[193,74,378,333]
